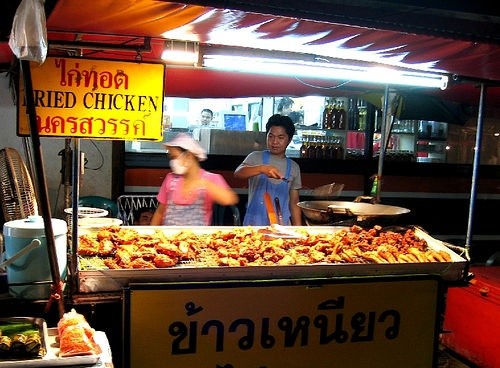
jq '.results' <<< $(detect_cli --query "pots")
[296,195,411,224]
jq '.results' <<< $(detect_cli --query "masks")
[169,152,187,174]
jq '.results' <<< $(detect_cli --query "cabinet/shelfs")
[288,96,368,156]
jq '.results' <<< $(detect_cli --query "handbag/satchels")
[8,0,48,65]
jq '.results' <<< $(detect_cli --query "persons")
[278,97,303,124]
[234,114,302,226]
[201,109,213,124]
[149,135,239,226]
[134,207,156,226]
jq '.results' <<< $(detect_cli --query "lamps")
[158,38,199,65]
[198,43,449,92]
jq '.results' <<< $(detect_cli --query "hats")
[160,133,207,161]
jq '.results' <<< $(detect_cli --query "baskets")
[64,207,108,249]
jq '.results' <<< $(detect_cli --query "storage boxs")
[195,127,267,154]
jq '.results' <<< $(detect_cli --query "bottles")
[322,100,329,130]
[391,119,416,133]
[298,106,304,124]
[348,98,367,132]
[329,136,344,160]
[374,107,383,133]
[334,101,340,130]
[315,136,329,159]
[327,99,333,130]
[330,100,336,129]
[338,101,346,130]
[300,135,315,159]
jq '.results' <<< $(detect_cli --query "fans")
[0,145,40,221]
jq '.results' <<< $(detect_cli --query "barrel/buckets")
[0,215,68,300]
